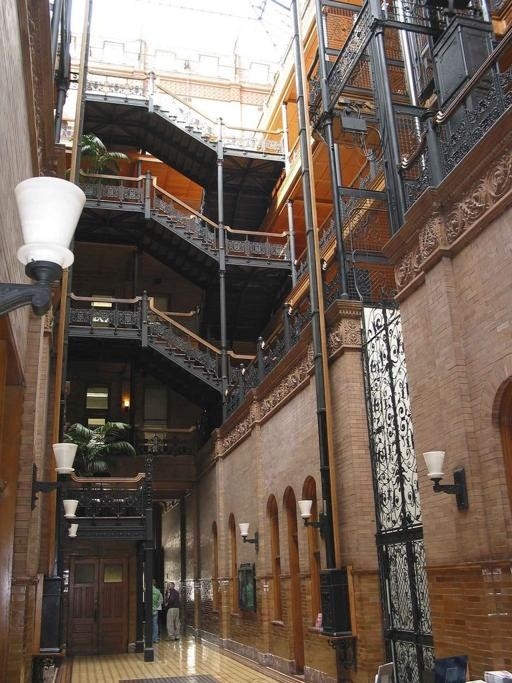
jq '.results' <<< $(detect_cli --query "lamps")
[298,499,326,539]
[32,442,80,548]
[0,174,87,317]
[238,522,258,549]
[422,448,469,512]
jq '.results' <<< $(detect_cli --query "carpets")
[118,674,221,682]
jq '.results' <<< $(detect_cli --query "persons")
[165,582,180,641]
[151,579,160,643]
[156,585,163,635]
